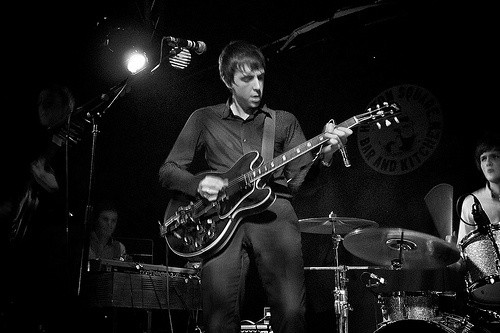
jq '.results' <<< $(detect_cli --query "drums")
[461,222,500,301]
[372,291,475,333]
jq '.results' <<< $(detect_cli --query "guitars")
[160,99,402,257]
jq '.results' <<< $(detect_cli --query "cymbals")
[343,227,460,270]
[296,216,379,235]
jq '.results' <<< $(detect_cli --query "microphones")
[473,197,492,227]
[166,36,207,54]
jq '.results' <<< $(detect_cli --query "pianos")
[71,258,205,333]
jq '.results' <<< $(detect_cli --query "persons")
[457,142,500,282]
[0,82,126,333]
[159,42,348,333]
